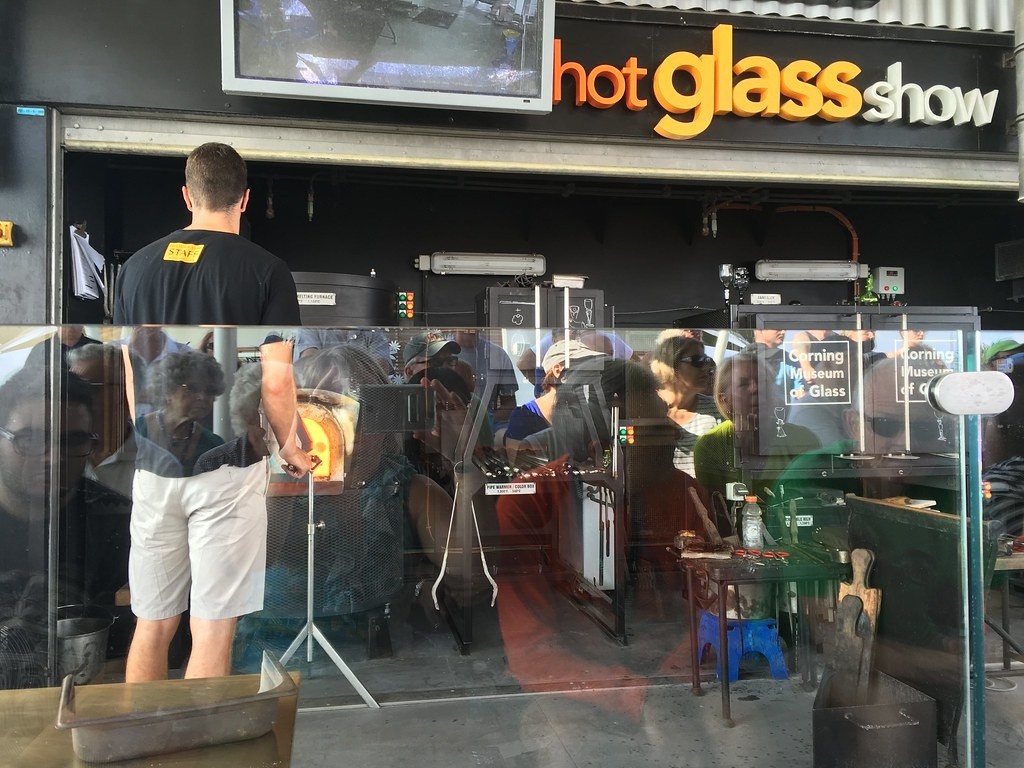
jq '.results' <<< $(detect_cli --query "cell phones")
[355,384,436,433]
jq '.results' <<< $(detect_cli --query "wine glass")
[734,266,749,305]
[719,264,733,309]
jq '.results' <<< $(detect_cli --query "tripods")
[278,470,380,707]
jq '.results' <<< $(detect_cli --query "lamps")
[414,253,547,276]
[754,260,869,281]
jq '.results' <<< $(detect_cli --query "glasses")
[80,380,120,392]
[297,395,356,415]
[177,382,220,396]
[678,354,716,368]
[5,424,100,461]
[856,409,956,440]
[204,343,215,351]
[410,356,459,370]
[990,352,1024,360]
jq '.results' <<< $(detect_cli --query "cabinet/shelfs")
[671,304,979,600]
[433,286,631,656]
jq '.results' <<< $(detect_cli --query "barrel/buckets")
[55,603,118,687]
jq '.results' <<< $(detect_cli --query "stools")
[698,609,790,684]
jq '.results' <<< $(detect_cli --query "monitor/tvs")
[220,0,555,115]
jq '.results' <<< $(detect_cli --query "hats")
[808,333,877,363]
[982,338,1024,364]
[403,330,462,367]
[542,340,607,374]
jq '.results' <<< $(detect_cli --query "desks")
[0,669,299,768]
[681,539,842,729]
[983,550,1024,679]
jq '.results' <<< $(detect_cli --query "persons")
[0,328,1024,693]
[110,142,313,686]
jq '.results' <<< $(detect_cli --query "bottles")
[741,496,765,550]
